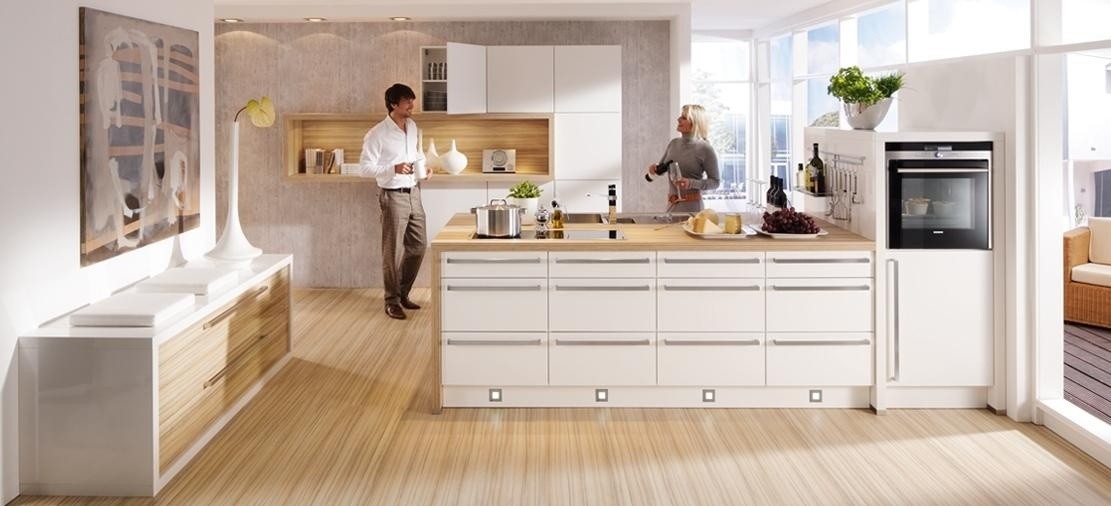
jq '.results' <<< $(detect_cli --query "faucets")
[653,212,673,224]
[551,200,569,221]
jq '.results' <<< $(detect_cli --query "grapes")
[761,207,821,234]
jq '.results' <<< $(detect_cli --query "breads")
[692,209,723,233]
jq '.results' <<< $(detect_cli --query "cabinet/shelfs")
[552,114,624,215]
[419,38,488,115]
[17,253,295,501]
[418,177,553,250]
[764,250,875,410]
[281,110,552,183]
[549,247,656,409]
[487,41,624,114]
[656,249,765,409]
[440,250,549,408]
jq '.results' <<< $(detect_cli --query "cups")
[412,160,427,179]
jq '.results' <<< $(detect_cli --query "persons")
[359,82,432,321]
[648,104,721,214]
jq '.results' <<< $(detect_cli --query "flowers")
[232,97,277,128]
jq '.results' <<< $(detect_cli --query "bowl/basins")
[933,202,957,217]
[906,202,929,215]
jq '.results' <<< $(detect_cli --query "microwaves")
[885,141,995,249]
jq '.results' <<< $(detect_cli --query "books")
[305,147,361,175]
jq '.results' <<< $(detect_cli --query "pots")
[471,197,526,238]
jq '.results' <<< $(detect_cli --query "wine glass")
[668,162,685,204]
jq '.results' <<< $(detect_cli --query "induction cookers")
[471,228,625,241]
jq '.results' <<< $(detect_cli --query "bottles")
[646,161,673,183]
[443,138,468,174]
[805,143,826,193]
[427,137,441,172]
[797,162,806,190]
[608,183,620,226]
[537,201,564,229]
[766,175,787,212]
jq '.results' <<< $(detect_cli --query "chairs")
[1064,215,1111,332]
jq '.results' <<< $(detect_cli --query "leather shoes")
[400,296,420,309]
[384,303,407,320]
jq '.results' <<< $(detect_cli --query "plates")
[749,222,829,240]
[681,221,758,238]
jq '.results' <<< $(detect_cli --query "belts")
[382,187,411,195]
[668,192,702,202]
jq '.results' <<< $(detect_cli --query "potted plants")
[507,176,546,228]
[826,64,916,131]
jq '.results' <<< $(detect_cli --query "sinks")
[633,212,695,224]
[604,216,635,224]
[561,213,604,224]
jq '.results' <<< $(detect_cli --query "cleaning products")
[608,183,617,225]
[552,204,564,228]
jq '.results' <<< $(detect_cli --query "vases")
[417,126,470,175]
[201,120,263,267]
